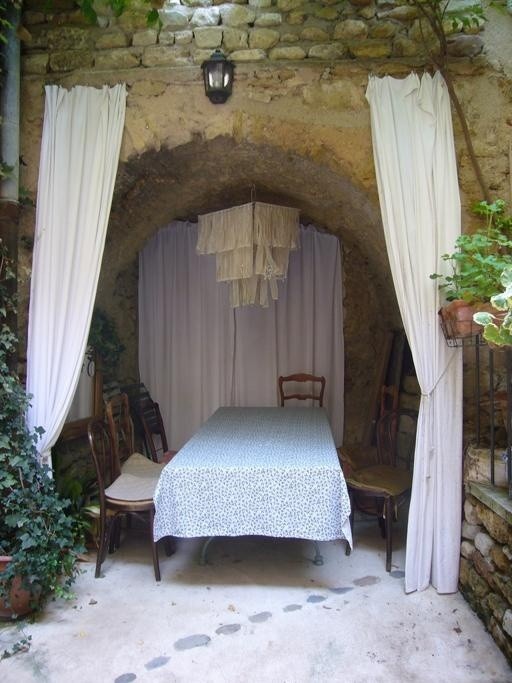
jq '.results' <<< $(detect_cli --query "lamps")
[196,182,303,311]
[199,47,237,105]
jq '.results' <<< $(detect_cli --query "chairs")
[278,372,326,408]
[87,380,179,583]
[345,407,419,572]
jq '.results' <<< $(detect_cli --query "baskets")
[440,319,487,348]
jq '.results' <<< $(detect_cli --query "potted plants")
[429,197,512,354]
[0,233,89,663]
